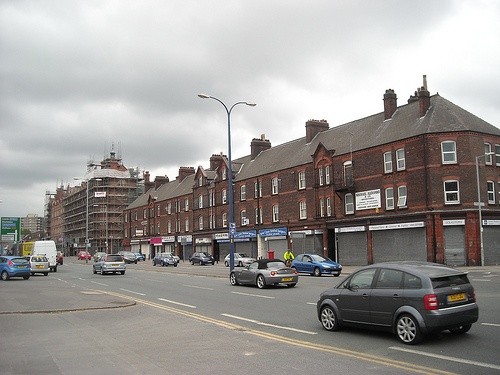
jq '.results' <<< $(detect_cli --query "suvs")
[23,254,51,276]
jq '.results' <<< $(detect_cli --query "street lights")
[475,151,496,267]
[197,94,256,274]
[73,177,103,253]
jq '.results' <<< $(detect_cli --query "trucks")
[0,239,58,272]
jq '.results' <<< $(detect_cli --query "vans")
[117,251,137,264]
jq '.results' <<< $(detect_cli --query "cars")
[77,251,91,260]
[224,253,257,267]
[169,252,180,263]
[92,254,126,276]
[94,252,106,262]
[230,258,298,290]
[289,254,342,277]
[0,255,31,281]
[56,251,63,265]
[134,253,145,261]
[188,252,215,266]
[316,261,480,345]
[152,253,177,267]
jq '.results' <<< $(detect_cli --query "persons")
[283,248,295,265]
